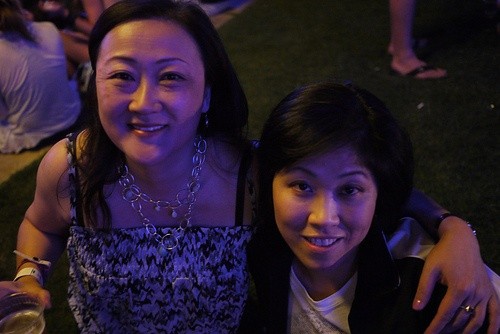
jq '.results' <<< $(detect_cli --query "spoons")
[0,291,46,334]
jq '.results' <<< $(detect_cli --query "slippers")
[388,64,448,80]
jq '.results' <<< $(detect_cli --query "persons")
[0,1,85,156]
[38,0,133,89]
[0,0,499,333]
[235,80,500,333]
[385,0,448,79]
[459,303,475,315]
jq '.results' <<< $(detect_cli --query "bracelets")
[12,268,52,288]
[431,210,459,241]
[12,250,52,275]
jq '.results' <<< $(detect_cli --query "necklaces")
[110,130,213,254]
